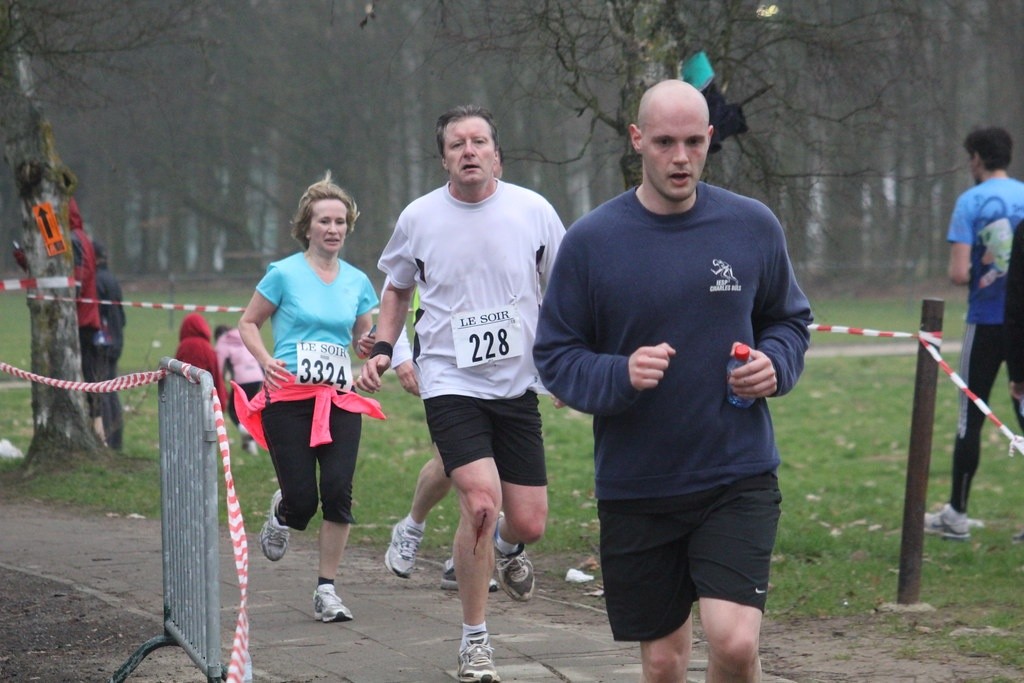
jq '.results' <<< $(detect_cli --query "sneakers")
[384,520,423,580]
[923,512,972,539]
[259,488,291,563]
[440,559,500,592]
[1012,528,1024,544]
[457,630,501,683]
[490,511,536,601]
[311,589,354,622]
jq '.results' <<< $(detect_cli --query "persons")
[238,167,378,623]
[356,103,567,683]
[64,198,126,453]
[385,144,502,592]
[533,78,814,683]
[176,315,265,457]
[924,126,1024,543]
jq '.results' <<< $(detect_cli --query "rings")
[358,375,365,379]
[271,372,274,376]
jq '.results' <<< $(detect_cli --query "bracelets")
[357,344,367,356]
[370,342,394,360]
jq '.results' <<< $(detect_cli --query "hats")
[91,240,106,260]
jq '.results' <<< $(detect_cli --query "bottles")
[728,345,756,408]
